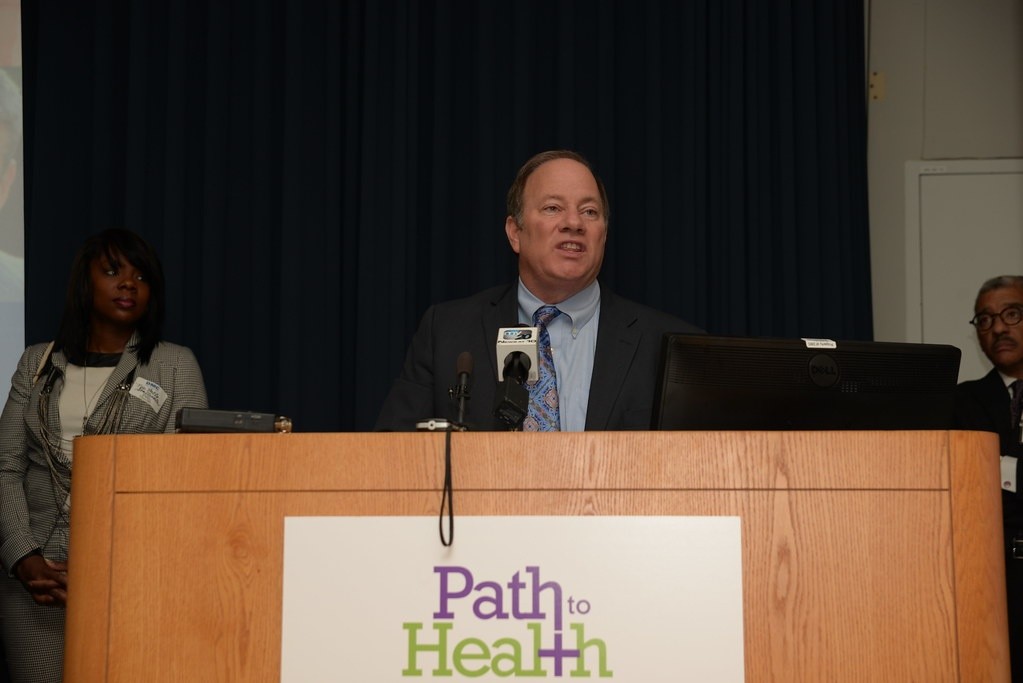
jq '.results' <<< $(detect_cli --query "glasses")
[970,306,1023,331]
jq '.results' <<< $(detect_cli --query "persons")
[0,222,208,683]
[952,275,1023,683]
[371,150,707,432]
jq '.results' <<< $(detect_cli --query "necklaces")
[36,366,136,526]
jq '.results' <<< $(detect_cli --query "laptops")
[649,332,961,432]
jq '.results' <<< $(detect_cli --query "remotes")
[415,418,470,432]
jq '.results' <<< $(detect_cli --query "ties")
[521,306,562,431]
[1011,380,1023,439]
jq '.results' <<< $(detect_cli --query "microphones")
[492,324,541,429]
[453,351,474,432]
[449,387,456,401]
